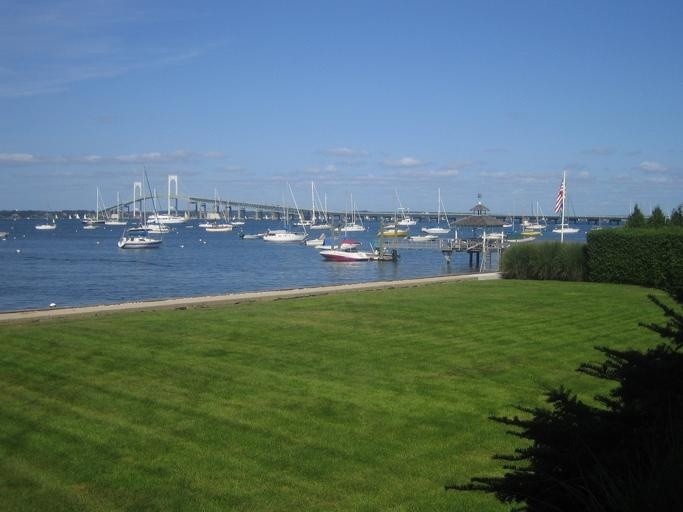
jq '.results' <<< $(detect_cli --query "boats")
[35,224,57,230]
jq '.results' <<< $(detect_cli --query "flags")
[555,169,567,219]
